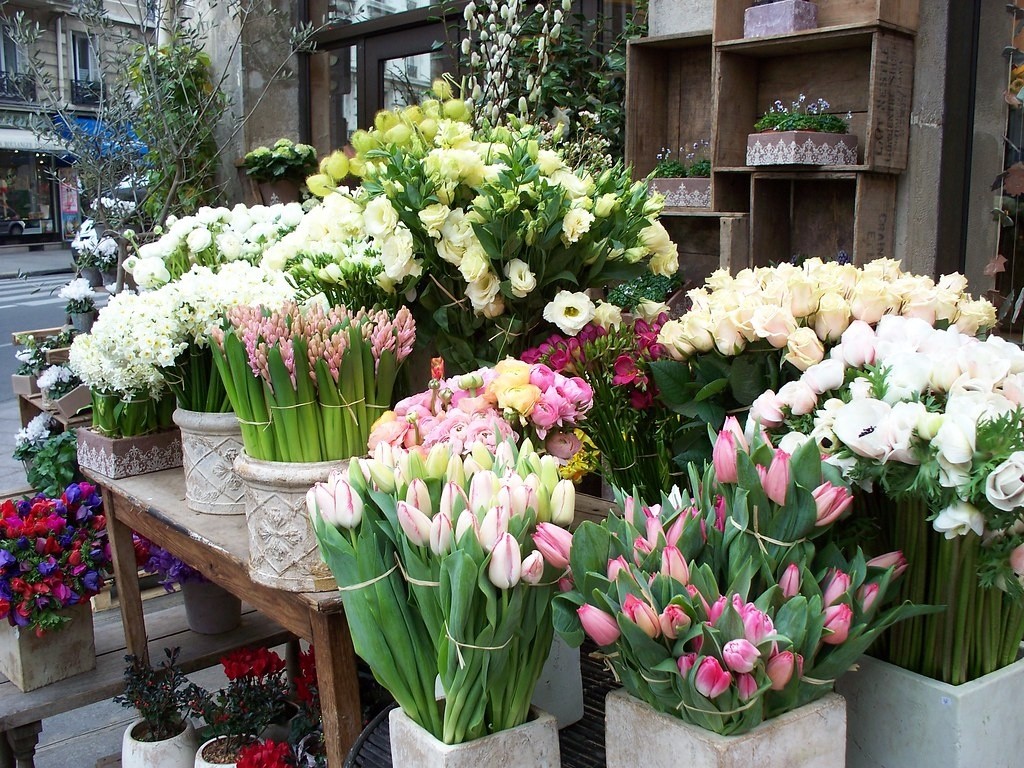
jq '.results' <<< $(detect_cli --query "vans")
[68,168,164,264]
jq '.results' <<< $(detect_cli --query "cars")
[0,205,26,235]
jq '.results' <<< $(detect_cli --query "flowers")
[0,1,1024,768]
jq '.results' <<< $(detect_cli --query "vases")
[11,373,42,394]
[297,731,328,768]
[641,178,710,207]
[258,701,300,744]
[743,1,819,41]
[388,695,560,768]
[233,447,368,592]
[834,641,1024,768]
[121,714,199,768]
[172,403,245,515]
[77,426,183,480]
[70,311,93,334]
[52,383,92,419]
[605,687,846,767]
[746,133,858,166]
[194,734,265,768]
[178,581,241,634]
[0,599,97,693]
[435,629,584,730]
